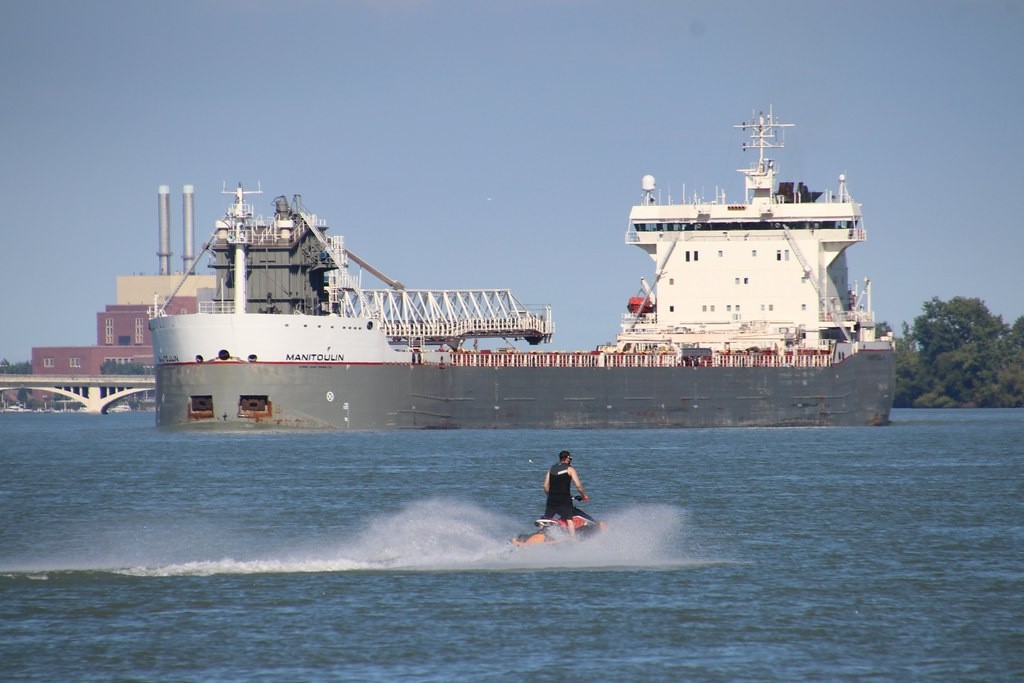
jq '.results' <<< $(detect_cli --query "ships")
[148,100,899,437]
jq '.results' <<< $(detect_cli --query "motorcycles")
[511,494,608,548]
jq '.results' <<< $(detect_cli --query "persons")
[542,451,589,539]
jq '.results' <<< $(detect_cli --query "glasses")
[570,457,572,460]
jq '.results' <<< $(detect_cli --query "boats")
[627,295,650,316]
